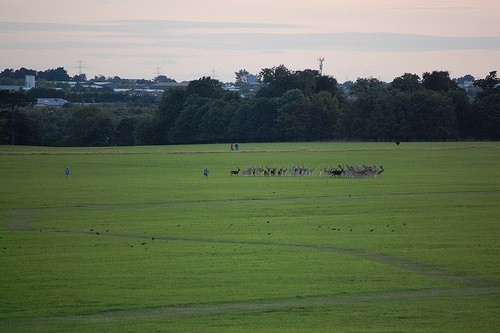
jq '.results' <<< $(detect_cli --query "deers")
[242,163,385,179]
[230,166,241,176]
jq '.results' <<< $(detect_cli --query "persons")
[231,144,240,151]
[202,168,209,180]
[64,167,70,183]
[394,138,400,151]
